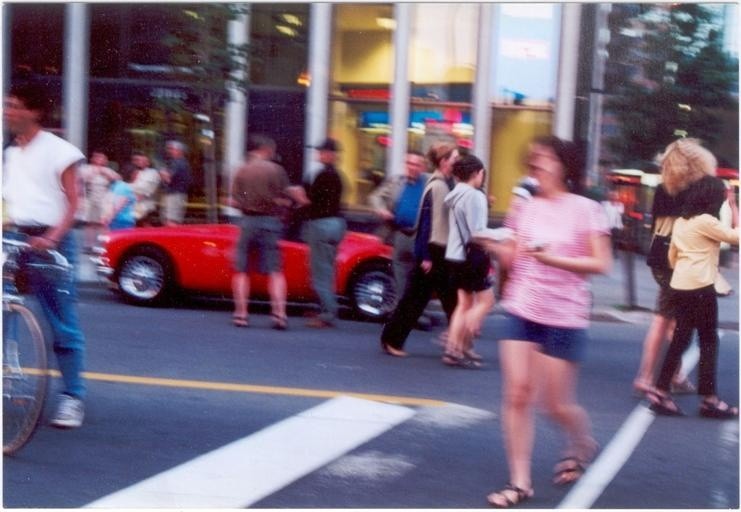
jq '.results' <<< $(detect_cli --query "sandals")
[487,482,535,507]
[698,395,738,420]
[434,332,489,371]
[231,312,290,331]
[634,374,695,418]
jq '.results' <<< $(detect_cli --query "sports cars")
[95,190,503,334]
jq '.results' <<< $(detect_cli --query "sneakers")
[51,394,87,431]
[304,310,335,329]
[380,341,409,358]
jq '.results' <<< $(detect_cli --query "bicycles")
[1,225,76,461]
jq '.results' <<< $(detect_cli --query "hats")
[306,135,342,152]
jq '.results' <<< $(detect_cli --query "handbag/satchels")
[457,241,493,293]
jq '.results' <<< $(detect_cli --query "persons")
[632,138,738,418]
[473,136,616,507]
[363,141,498,369]
[4,82,87,428]
[231,137,347,327]
[80,138,194,237]
